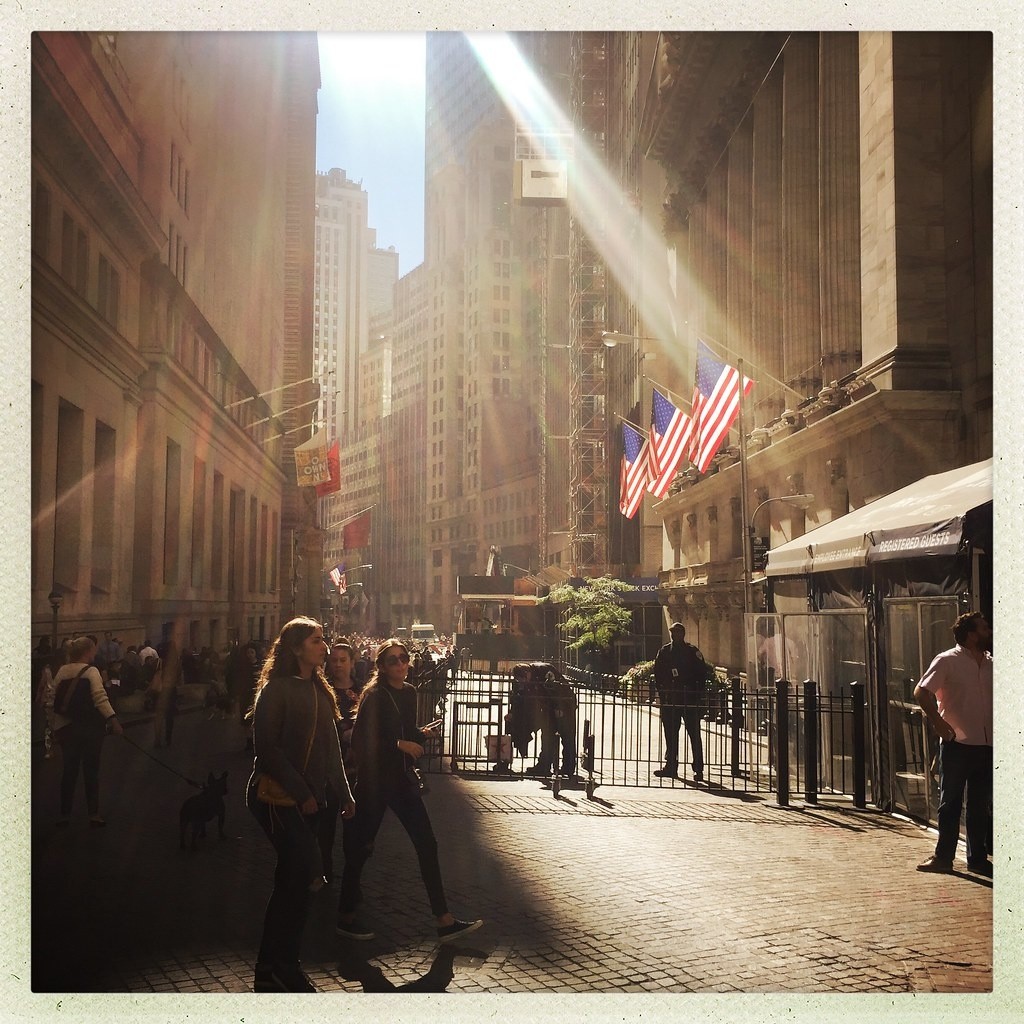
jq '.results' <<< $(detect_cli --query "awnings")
[764,457,992,578]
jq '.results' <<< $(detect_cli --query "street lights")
[602,326,818,730]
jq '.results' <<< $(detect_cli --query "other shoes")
[916,855,953,873]
[272,957,316,993]
[693,770,703,781]
[967,859,993,879]
[55,815,72,827]
[561,762,575,774]
[255,970,287,992]
[527,765,550,775]
[89,818,105,827]
[654,767,677,777]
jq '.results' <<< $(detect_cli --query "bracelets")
[397,739,400,750]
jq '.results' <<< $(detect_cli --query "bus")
[411,624,435,645]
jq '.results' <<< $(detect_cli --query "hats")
[144,640,152,645]
[669,622,685,631]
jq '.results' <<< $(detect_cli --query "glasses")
[384,654,410,666]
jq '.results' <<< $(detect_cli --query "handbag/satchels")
[53,665,93,720]
[403,764,426,796]
[143,689,160,713]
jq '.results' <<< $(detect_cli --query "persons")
[32,617,483,993]
[654,622,706,781]
[513,662,578,776]
[745,622,798,685]
[913,610,993,878]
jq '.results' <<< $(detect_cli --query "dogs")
[179,771,228,840]
[204,679,236,721]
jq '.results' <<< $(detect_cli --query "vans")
[397,627,407,638]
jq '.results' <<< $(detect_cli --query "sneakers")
[336,919,376,940]
[437,918,483,943]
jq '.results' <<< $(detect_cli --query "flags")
[619,336,755,518]
[294,424,372,595]
